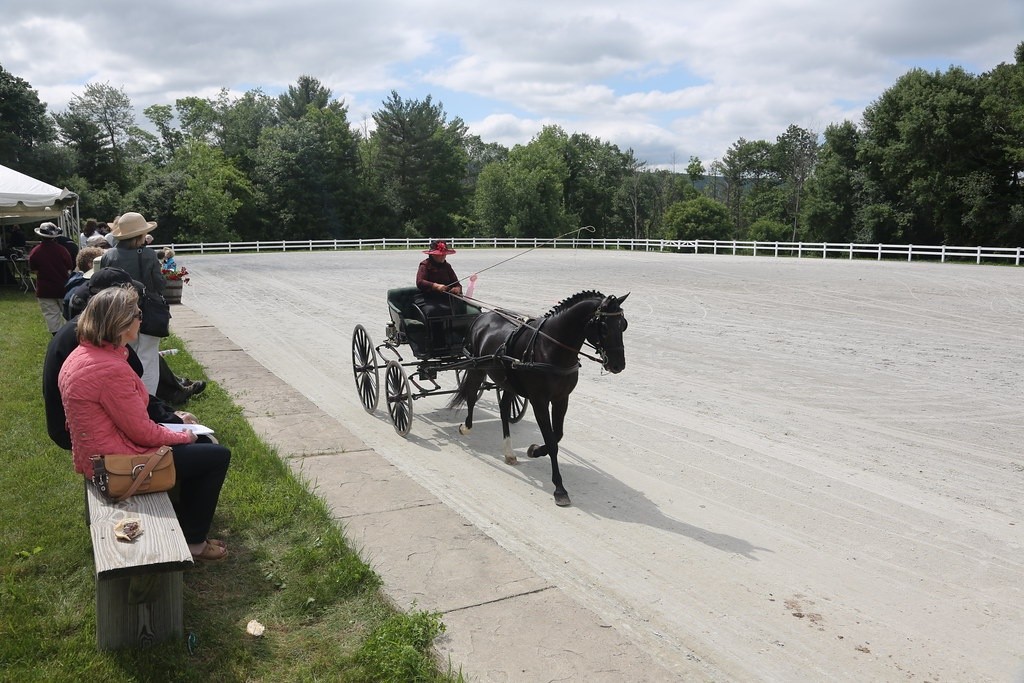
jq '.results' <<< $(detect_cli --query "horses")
[444,291,631,507]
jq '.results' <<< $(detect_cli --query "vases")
[162,280,183,304]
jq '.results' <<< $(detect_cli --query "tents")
[0,165,82,252]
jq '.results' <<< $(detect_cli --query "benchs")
[84,472,194,650]
[386,287,481,342]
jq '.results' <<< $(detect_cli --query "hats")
[107,215,120,230]
[111,212,158,241]
[89,266,147,290]
[422,239,456,255]
[34,221,64,238]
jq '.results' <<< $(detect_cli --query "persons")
[11,212,233,561]
[414,240,462,316]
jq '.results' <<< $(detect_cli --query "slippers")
[190,543,228,561]
[205,537,228,550]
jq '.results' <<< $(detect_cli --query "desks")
[0,255,31,280]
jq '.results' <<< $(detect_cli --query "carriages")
[352,286,628,508]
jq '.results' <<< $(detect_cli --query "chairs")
[11,253,37,295]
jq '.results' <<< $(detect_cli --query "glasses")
[133,310,143,320]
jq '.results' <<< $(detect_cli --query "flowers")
[161,267,191,284]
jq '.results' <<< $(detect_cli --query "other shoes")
[184,380,206,399]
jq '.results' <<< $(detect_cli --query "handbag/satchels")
[89,444,177,503]
[63,240,79,271]
[138,292,172,337]
